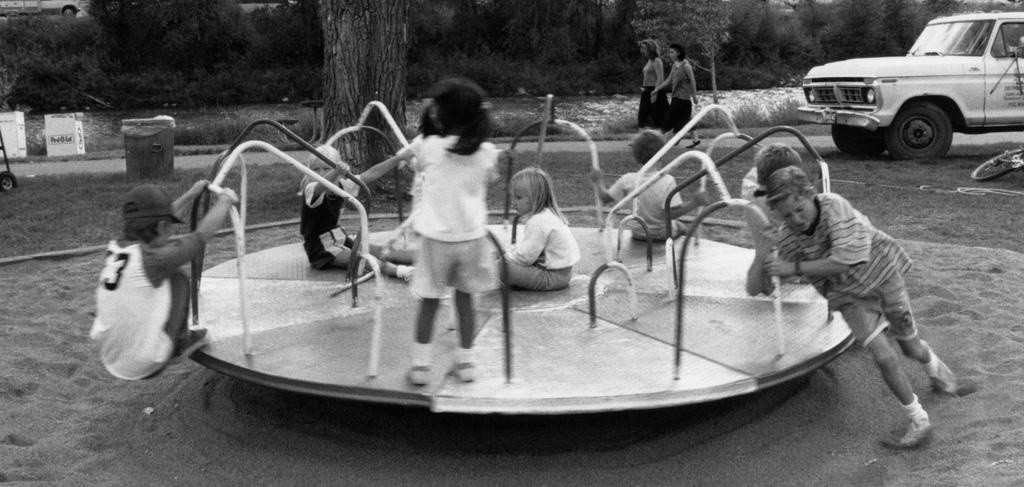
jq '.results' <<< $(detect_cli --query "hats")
[120,182,186,224]
[764,164,815,205]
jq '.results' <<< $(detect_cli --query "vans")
[0,0,82,18]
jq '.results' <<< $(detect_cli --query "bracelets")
[795,260,802,277]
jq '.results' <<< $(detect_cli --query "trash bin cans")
[121,119,174,183]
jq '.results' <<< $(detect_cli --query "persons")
[88,179,238,385]
[591,131,709,243]
[401,101,462,241]
[740,148,824,296]
[630,39,671,141]
[496,167,581,292]
[745,169,956,448]
[300,141,429,282]
[649,44,702,148]
[406,78,496,385]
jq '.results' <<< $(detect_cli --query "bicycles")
[968,147,1024,183]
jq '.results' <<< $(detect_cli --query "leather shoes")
[686,140,700,148]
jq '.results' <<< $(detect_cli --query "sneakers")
[168,327,209,365]
[899,411,931,448]
[920,339,957,395]
[452,356,478,383]
[407,363,433,387]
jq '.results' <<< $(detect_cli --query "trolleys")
[0,125,18,193]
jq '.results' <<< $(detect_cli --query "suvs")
[794,7,1024,161]
